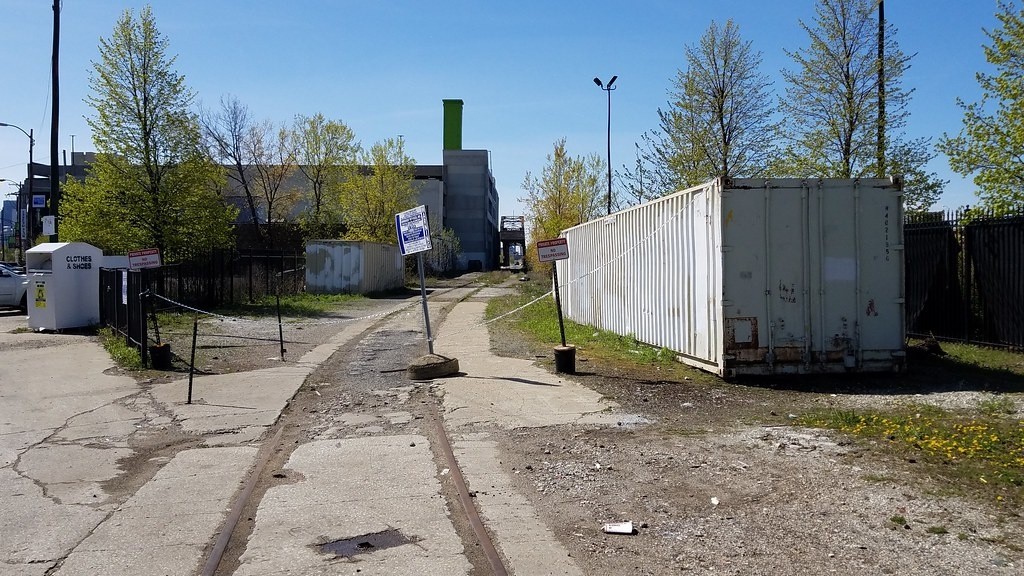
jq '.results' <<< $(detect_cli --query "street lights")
[0,123,33,251]
[594,75,620,214]
[0,178,22,262]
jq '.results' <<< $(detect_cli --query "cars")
[0,262,30,316]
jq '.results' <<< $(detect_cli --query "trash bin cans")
[25,241,104,333]
